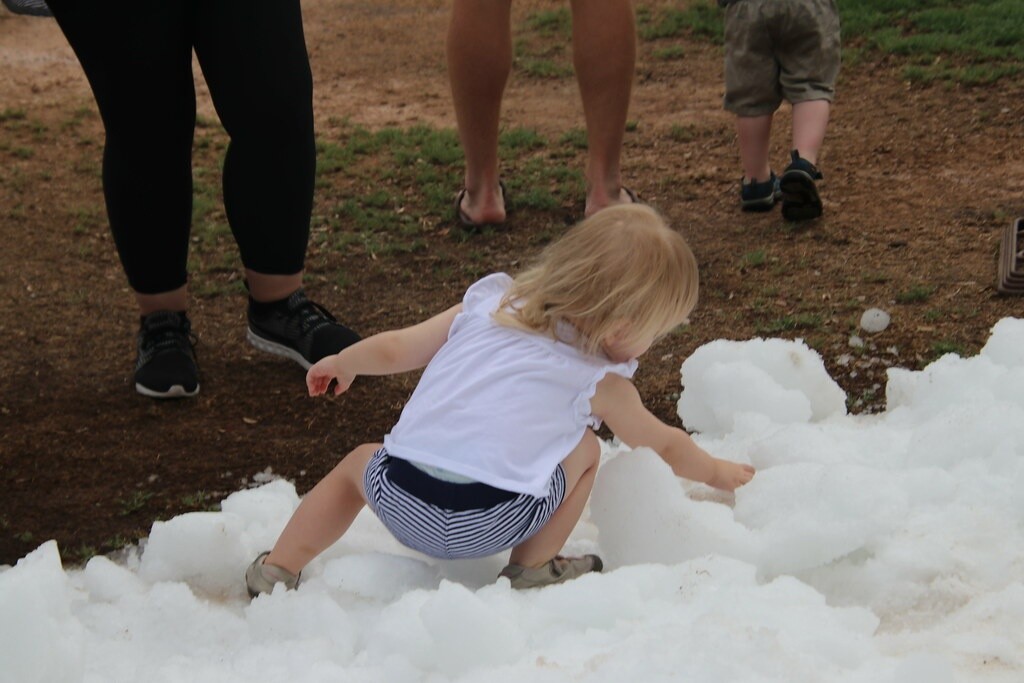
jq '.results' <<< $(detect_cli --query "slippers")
[574,184,650,225]
[453,178,514,228]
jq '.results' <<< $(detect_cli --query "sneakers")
[245,551,303,599]
[242,277,365,381]
[739,169,778,210]
[778,148,824,220]
[131,318,202,399]
[496,555,604,591]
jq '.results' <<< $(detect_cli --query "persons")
[719,1,841,223]
[247,204,755,600]
[447,0,640,234]
[4,0,359,399]
